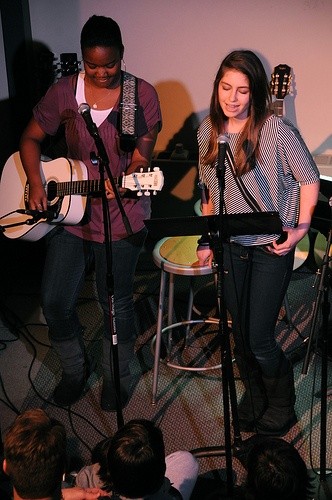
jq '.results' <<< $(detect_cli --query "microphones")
[77,103,99,136]
[217,133,228,170]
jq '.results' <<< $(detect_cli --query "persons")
[231,438,315,500]
[19,14,163,412]
[0,406,199,500]
[196,50,320,438]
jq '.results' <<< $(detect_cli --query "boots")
[234,347,268,432]
[254,363,296,435]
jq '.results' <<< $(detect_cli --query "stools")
[149,236,236,406]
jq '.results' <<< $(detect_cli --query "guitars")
[55,52,82,74]
[0,148,165,240]
[268,63,296,119]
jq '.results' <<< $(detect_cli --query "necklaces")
[87,83,112,110]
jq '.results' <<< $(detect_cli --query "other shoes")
[99,377,125,412]
[53,369,91,406]
